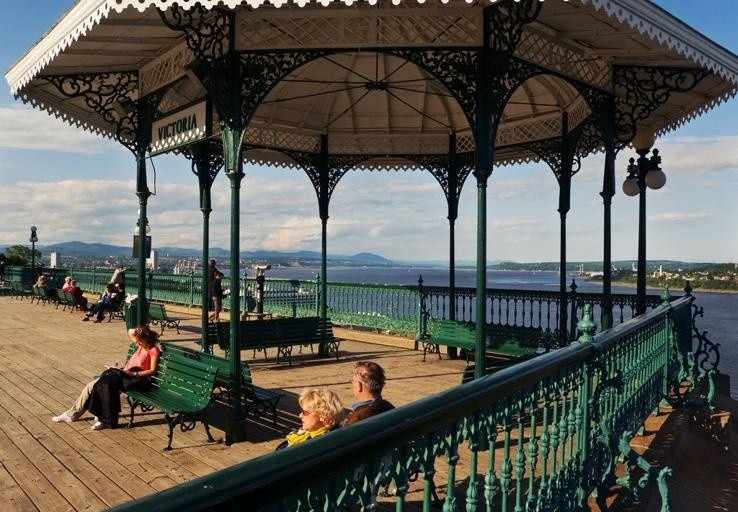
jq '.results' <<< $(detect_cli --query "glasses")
[301,409,313,416]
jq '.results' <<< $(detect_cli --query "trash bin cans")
[124,302,137,333]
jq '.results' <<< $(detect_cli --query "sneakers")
[91,416,107,430]
[51,411,75,423]
[82,312,102,323]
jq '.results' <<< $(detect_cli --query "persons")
[83,325,160,430]
[207,258,225,311]
[34,263,129,324]
[50,326,139,425]
[270,387,348,453]
[207,272,228,324]
[334,359,397,422]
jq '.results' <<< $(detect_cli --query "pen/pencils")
[116,362,118,367]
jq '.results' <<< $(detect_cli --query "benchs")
[457,359,515,384]
[193,316,321,363]
[419,317,541,369]
[9,280,181,337]
[216,317,344,369]
[158,340,284,442]
[118,341,219,453]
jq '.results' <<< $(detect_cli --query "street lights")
[135,206,151,234]
[29,226,38,285]
[622,118,666,317]
